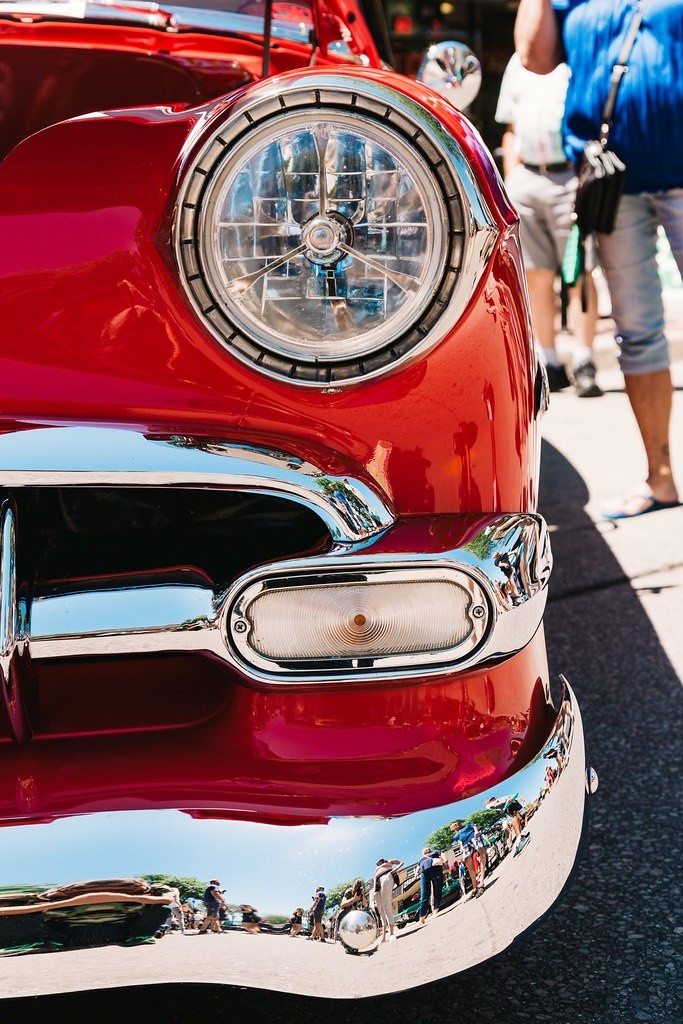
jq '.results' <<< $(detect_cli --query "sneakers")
[546,363,571,391]
[574,361,604,397]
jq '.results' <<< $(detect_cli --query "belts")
[522,161,568,172]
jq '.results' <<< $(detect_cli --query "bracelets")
[222,901,223,902]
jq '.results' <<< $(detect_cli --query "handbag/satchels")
[573,140,629,234]
[583,233,600,271]
[561,223,582,285]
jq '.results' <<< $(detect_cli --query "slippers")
[602,496,683,519]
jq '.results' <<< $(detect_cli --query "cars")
[0,0,599,1007]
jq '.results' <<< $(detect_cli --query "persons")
[459,863,466,898]
[321,478,383,535]
[450,822,487,902]
[164,882,186,934]
[439,700,575,912]
[515,0,683,528]
[483,793,522,855]
[198,879,227,934]
[492,516,543,608]
[308,886,327,942]
[181,879,382,945]
[373,858,404,944]
[494,51,603,398]
[414,848,448,927]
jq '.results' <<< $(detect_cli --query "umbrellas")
[185,897,203,911]
